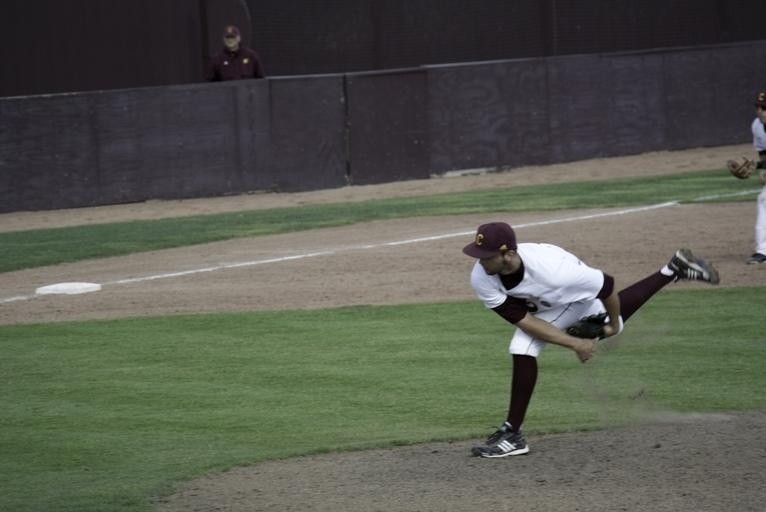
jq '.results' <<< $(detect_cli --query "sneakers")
[471,421,530,458]
[667,248,720,285]
[746,252,766,264]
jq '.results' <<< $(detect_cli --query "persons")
[202,25,264,83]
[462,222,719,459]
[727,89,766,265]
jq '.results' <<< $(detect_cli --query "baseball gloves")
[727,158,756,180]
[566,311,609,340]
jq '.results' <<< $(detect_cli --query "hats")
[751,90,766,105]
[223,26,241,38]
[463,221,517,260]
